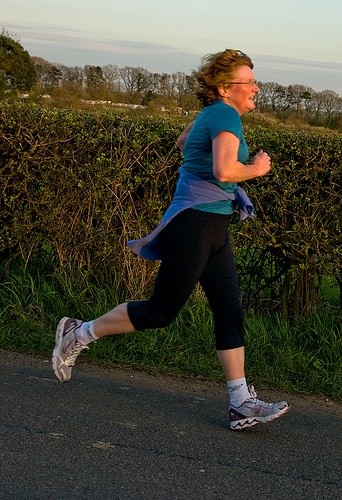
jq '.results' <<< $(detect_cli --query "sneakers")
[52,316,87,383]
[228,397,290,430]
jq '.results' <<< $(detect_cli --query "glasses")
[225,78,257,86]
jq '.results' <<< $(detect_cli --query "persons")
[51,48,291,430]
